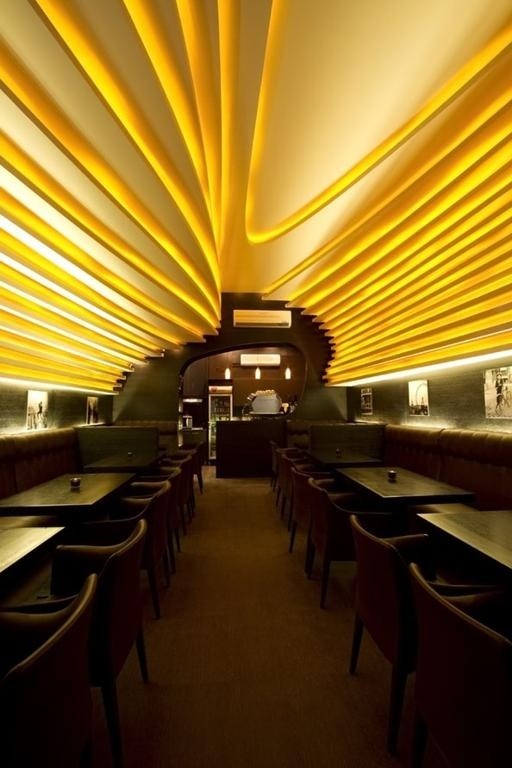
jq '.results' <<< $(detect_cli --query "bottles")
[408,395,428,415]
[178,432,184,448]
[178,414,183,431]
[186,416,193,430]
[283,394,298,416]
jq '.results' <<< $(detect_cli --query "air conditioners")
[233,309,293,328]
[240,353,281,367]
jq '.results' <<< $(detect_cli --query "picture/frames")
[483,364,512,421]
[407,379,430,416]
[86,396,99,425]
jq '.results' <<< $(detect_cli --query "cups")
[387,470,396,480]
[70,478,81,489]
[128,451,133,457]
[335,448,340,457]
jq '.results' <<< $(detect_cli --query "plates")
[183,428,191,431]
[250,411,284,418]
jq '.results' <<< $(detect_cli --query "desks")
[2,474,136,515]
[182,427,208,466]
[305,448,382,466]
[335,467,474,508]
[84,449,166,472]
[416,511,510,570]
[3,528,66,572]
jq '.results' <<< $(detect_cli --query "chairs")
[2,519,148,765]
[3,573,97,767]
[276,451,379,609]
[113,451,196,620]
[348,514,491,754]
[407,562,510,767]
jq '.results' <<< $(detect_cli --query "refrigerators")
[207,380,234,464]
